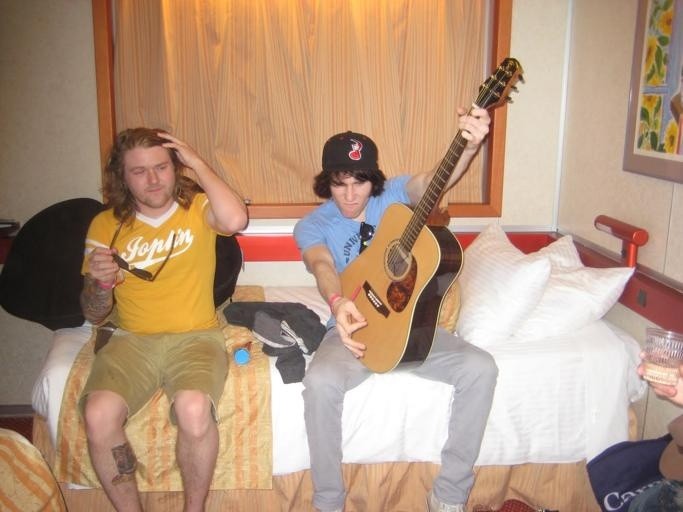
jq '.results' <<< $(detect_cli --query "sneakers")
[425,490,466,512]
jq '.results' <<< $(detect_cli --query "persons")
[293,104,497,512]
[635,342,683,404]
[78,125,248,512]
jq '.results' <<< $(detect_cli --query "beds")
[30,286,683,512]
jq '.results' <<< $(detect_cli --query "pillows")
[453,225,549,351]
[511,233,635,355]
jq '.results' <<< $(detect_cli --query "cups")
[645,327,682,386]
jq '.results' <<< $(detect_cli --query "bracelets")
[325,286,363,310]
[94,279,117,291]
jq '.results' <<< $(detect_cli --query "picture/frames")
[623,0,683,183]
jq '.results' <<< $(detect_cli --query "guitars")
[340,57,526,374]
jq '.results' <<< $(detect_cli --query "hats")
[321,130,378,172]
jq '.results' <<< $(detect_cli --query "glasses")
[108,215,176,283]
